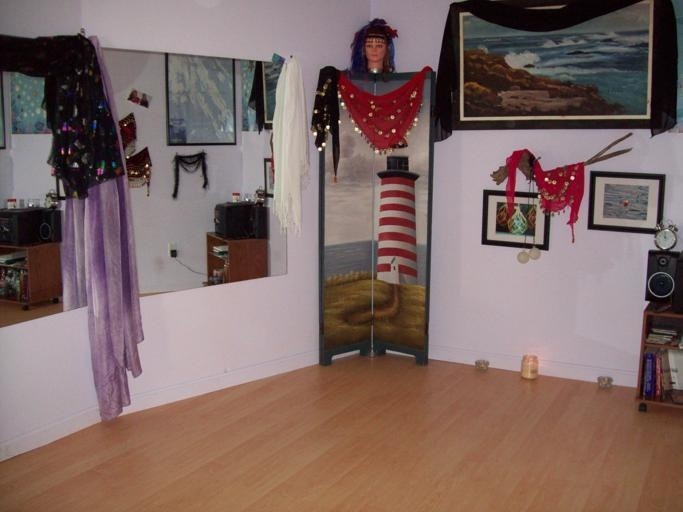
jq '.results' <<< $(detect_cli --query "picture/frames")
[480,189,550,250]
[448,2,661,131]
[588,169,666,234]
[260,61,283,129]
[163,54,238,147]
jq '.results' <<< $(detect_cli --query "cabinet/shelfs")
[1,243,61,309]
[635,305,682,411]
[203,231,271,286]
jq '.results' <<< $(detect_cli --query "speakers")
[0,208,62,246]
[215,201,268,239]
[645,249,681,303]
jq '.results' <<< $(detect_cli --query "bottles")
[6,199,16,211]
[15,275,21,300]
[232,192,240,204]
[521,355,539,379]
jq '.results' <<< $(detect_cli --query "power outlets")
[168,242,177,258]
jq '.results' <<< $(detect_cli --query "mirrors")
[93,45,290,294]
[0,62,93,327]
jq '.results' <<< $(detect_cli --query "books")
[642,327,683,398]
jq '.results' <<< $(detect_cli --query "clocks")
[655,229,676,250]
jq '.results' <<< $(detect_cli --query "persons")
[349,19,399,74]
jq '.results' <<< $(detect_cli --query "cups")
[244,193,256,205]
[598,376,614,390]
[474,361,490,370]
[27,198,40,207]
